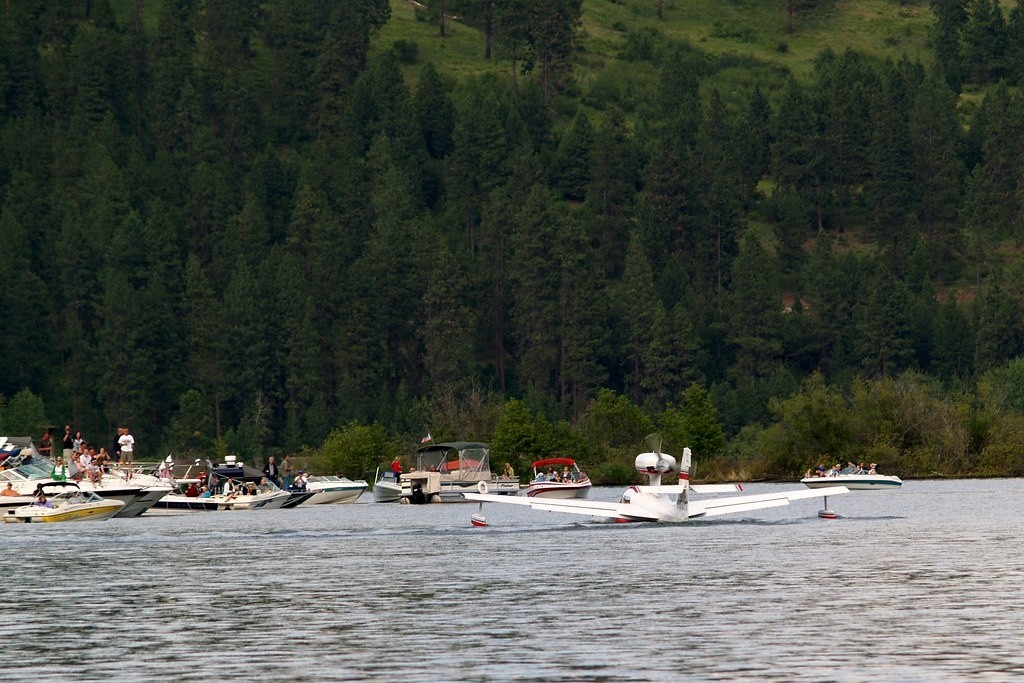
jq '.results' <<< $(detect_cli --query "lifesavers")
[478,480,488,494]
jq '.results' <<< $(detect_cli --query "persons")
[804,461,877,478]
[185,479,211,497]
[279,454,294,491]
[112,426,135,480]
[50,456,67,487]
[223,477,269,499]
[32,483,46,497]
[37,430,54,456]
[67,490,83,504]
[430,464,441,473]
[62,424,110,488]
[294,470,307,491]
[34,495,53,508]
[262,455,279,486]
[533,465,588,483]
[410,467,415,474]
[391,455,402,485]
[502,463,514,480]
[0,482,20,497]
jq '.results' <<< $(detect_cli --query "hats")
[298,470,304,474]
[870,463,877,467]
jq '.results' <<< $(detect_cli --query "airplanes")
[459,440,850,532]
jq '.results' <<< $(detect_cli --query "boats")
[525,458,592,499]
[799,476,902,490]
[287,475,369,506]
[3,482,125,526]
[154,455,290,509]
[373,442,520,504]
[0,435,201,518]
